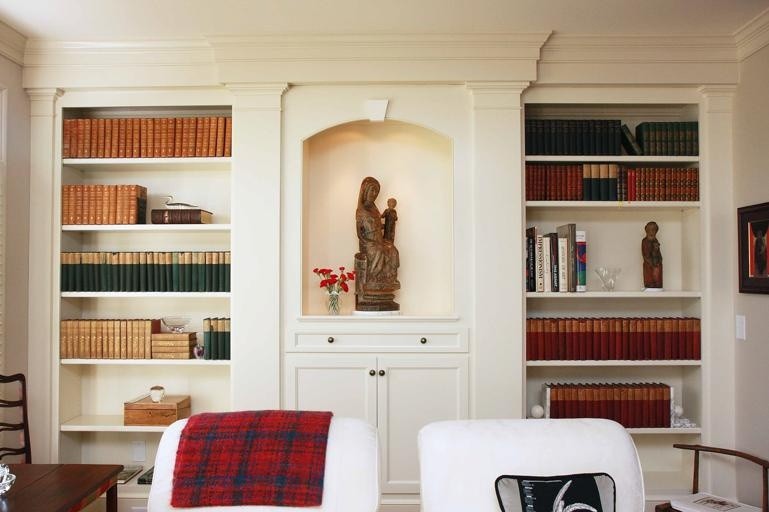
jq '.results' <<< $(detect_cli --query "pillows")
[492,469,620,512]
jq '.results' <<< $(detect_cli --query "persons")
[642,221,663,287]
[355,176,401,299]
[380,198,397,243]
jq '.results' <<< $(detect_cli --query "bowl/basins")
[162,313,192,334]
[0,473,16,495]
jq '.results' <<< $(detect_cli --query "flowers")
[311,265,358,312]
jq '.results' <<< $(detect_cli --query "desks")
[0,462,126,512]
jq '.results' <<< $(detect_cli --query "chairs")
[655,442,768,511]
[143,411,383,511]
[416,415,648,511]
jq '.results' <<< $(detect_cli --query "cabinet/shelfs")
[54,153,235,436]
[521,151,704,438]
[279,320,470,499]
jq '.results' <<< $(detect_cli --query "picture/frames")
[735,200,769,295]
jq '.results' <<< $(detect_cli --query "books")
[524,119,700,201]
[137,465,155,486]
[60,318,230,359]
[151,210,213,224]
[62,185,146,224]
[61,252,231,292]
[63,116,232,158]
[524,222,588,294]
[526,316,701,428]
[116,464,144,485]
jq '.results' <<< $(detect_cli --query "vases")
[322,286,347,316]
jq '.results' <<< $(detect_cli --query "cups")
[596,264,623,292]
[149,384,165,403]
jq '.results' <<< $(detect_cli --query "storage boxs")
[122,392,194,426]
